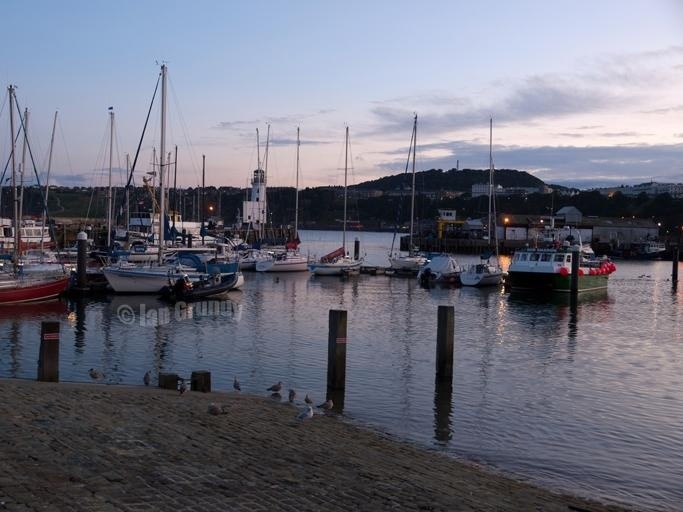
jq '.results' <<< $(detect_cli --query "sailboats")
[388,112,433,276]
[460,117,508,287]
[306,126,367,276]
[0,62,316,304]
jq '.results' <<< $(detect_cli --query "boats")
[609,233,666,259]
[327,218,364,231]
[417,250,464,285]
[504,226,616,303]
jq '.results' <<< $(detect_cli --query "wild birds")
[86,367,107,384]
[233,375,242,392]
[179,377,187,395]
[266,380,286,392]
[305,394,312,405]
[316,399,334,413]
[296,406,314,423]
[142,370,152,386]
[287,388,296,402]
